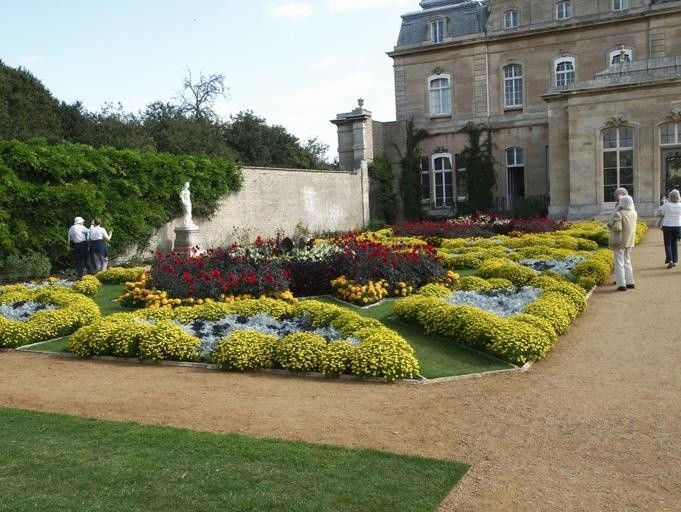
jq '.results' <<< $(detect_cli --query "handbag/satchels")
[608,227,622,246]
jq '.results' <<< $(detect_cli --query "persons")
[178,181,194,227]
[660,189,680,269]
[606,195,637,290]
[88,216,114,272]
[66,215,95,282]
[611,187,629,285]
[619,44,626,63]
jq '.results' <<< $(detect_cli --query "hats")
[619,195,634,211]
[74,217,85,224]
[614,188,629,201]
